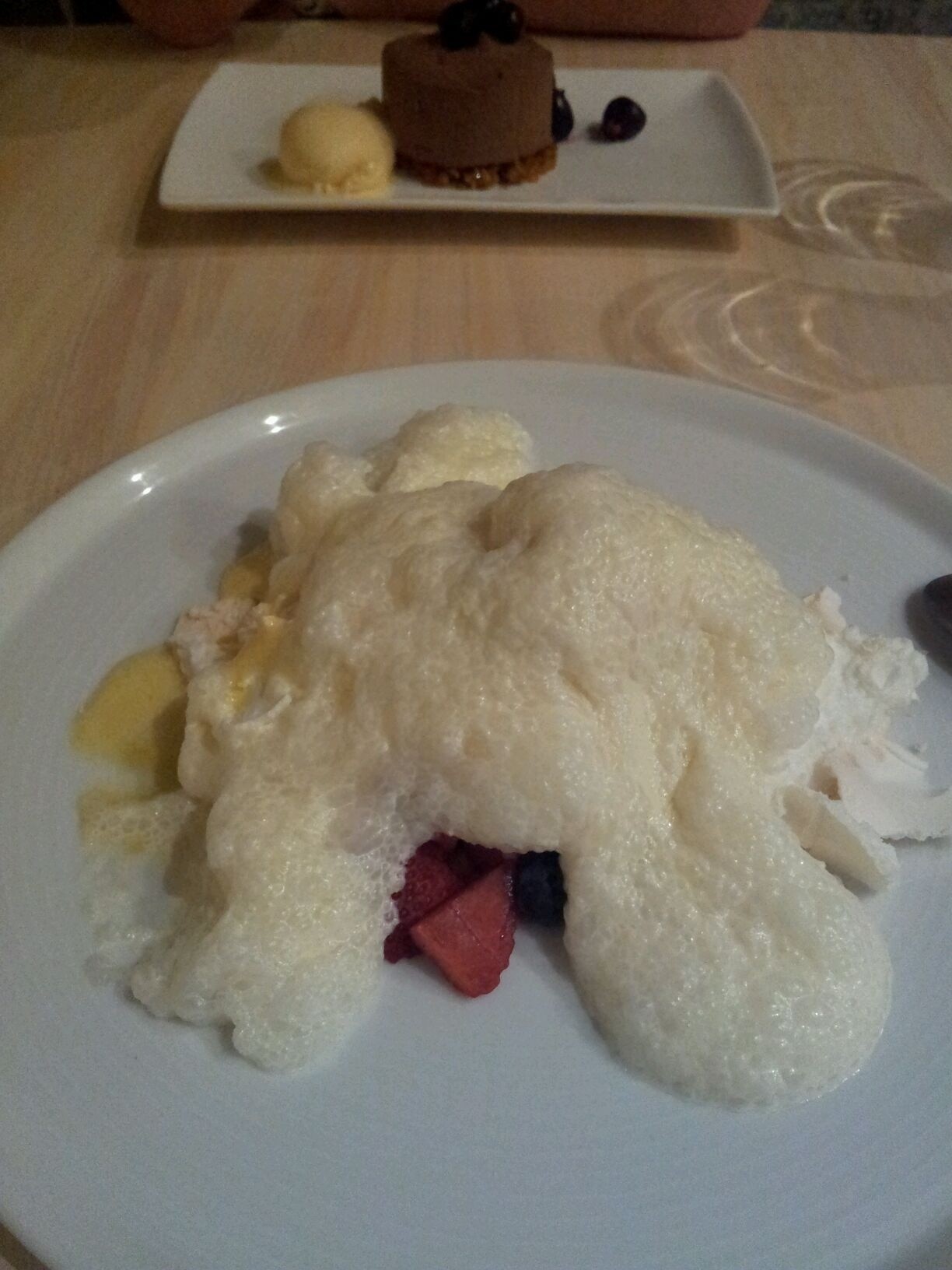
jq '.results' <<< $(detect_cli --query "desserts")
[380,0,555,185]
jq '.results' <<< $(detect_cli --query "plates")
[0,363,951,1269]
[161,62,780,223]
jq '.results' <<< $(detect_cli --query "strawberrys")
[383,832,521,995]
